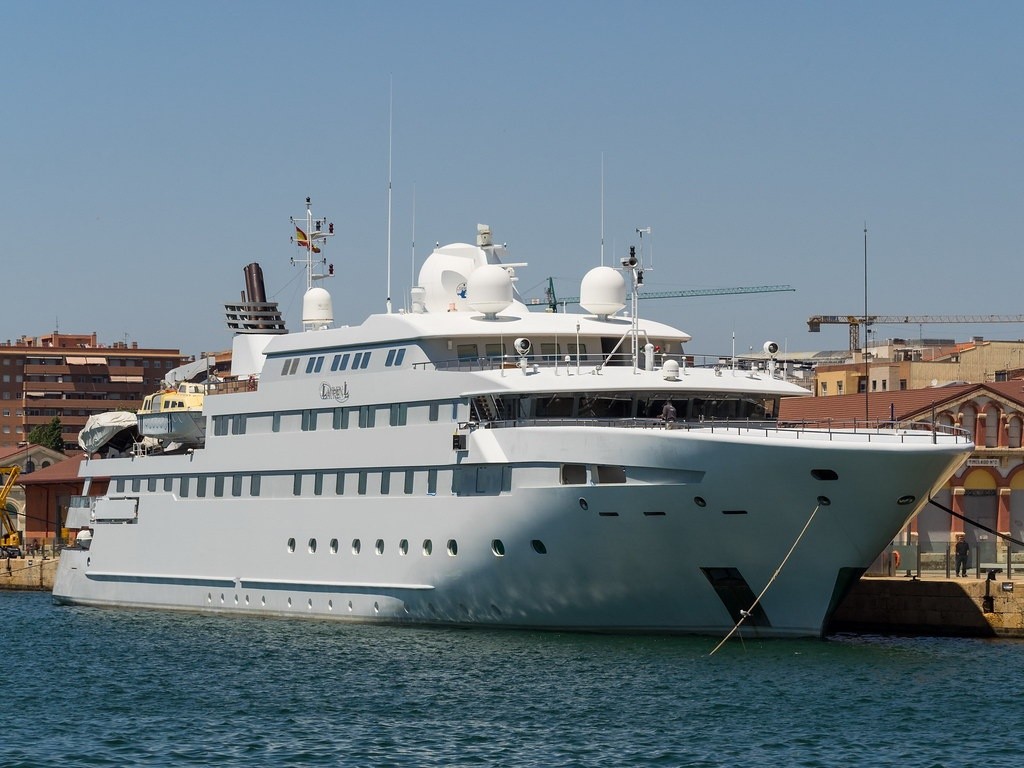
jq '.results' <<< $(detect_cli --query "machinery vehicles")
[0,464,24,558]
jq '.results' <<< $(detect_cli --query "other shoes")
[962,574,968,578]
[956,574,961,578]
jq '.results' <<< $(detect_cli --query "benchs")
[979,562,1024,573]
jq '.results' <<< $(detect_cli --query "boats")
[50,80,978,658]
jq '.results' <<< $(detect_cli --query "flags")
[296,226,321,253]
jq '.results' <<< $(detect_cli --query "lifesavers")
[891,550,901,569]
[247,376,256,391]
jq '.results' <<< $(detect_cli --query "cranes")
[523,274,795,314]
[802,314,1024,357]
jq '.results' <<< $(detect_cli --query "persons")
[32,539,40,550]
[955,536,969,576]
[661,401,677,421]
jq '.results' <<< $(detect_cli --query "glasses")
[960,537,965,540]
